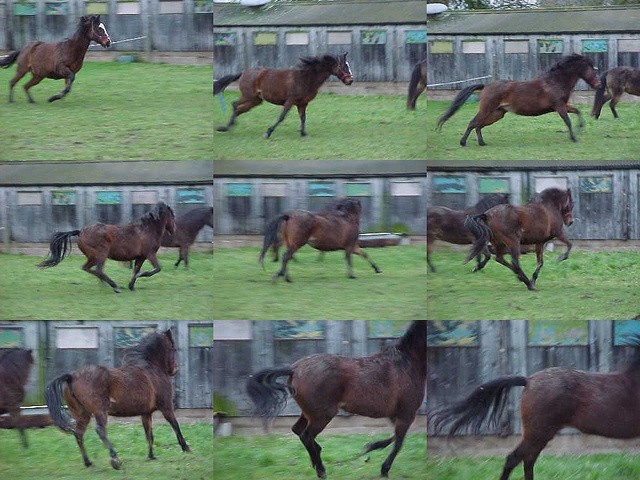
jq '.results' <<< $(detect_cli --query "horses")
[214,52,354,139]
[130,206,213,271]
[258,198,383,283]
[435,53,602,146]
[428,333,640,480]
[0,348,37,448]
[0,14,111,103]
[35,201,177,293]
[407,59,427,111]
[427,193,511,272]
[463,188,576,290]
[43,329,194,470]
[246,320,427,480]
[591,66,640,119]
